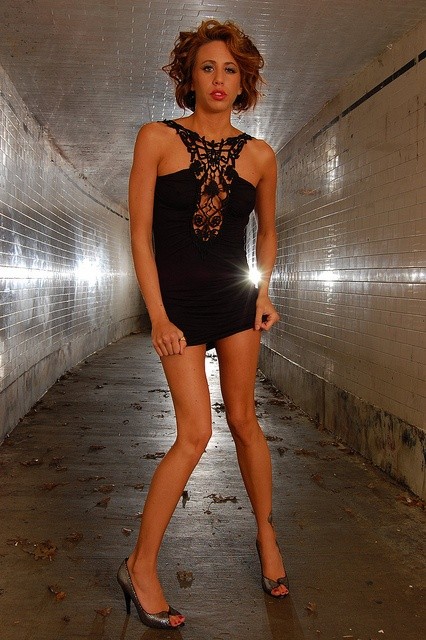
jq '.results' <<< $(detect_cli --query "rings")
[179,337,186,341]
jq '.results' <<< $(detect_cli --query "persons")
[117,19,292,632]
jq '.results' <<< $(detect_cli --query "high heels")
[255,538,290,597]
[116,558,186,631]
